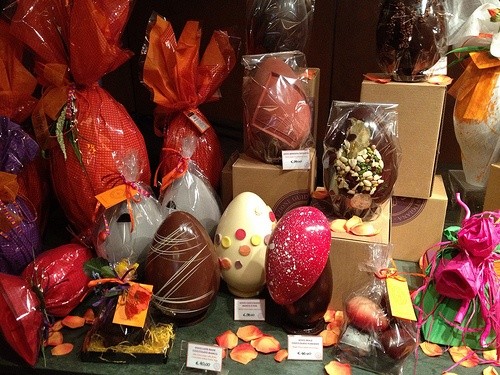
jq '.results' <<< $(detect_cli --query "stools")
[447,169,486,226]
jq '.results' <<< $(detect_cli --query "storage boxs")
[483,161,500,211]
[219,67,321,208]
[357,79,447,200]
[312,188,392,316]
[390,174,449,265]
